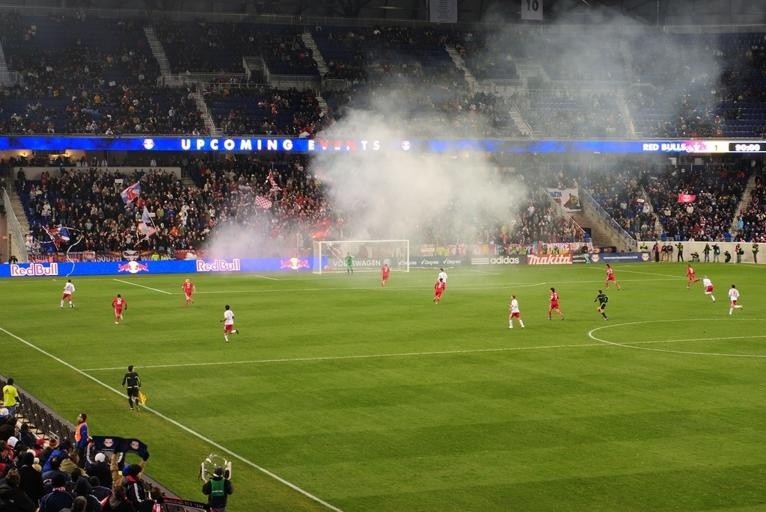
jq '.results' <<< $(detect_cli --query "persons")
[508,295,525,329]
[0,377,167,512]
[605,263,620,290]
[729,285,744,315]
[685,264,701,289]
[121,366,141,412]
[380,264,390,288]
[1,2,766,263]
[593,290,608,320]
[60,279,75,308]
[703,275,716,303]
[548,288,564,320]
[220,305,239,343]
[202,466,233,512]
[433,278,446,305]
[182,279,195,304]
[345,253,353,274]
[438,268,447,287]
[112,294,127,325]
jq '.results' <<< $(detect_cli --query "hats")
[94,452,106,463]
[51,473,66,489]
[121,464,142,478]
